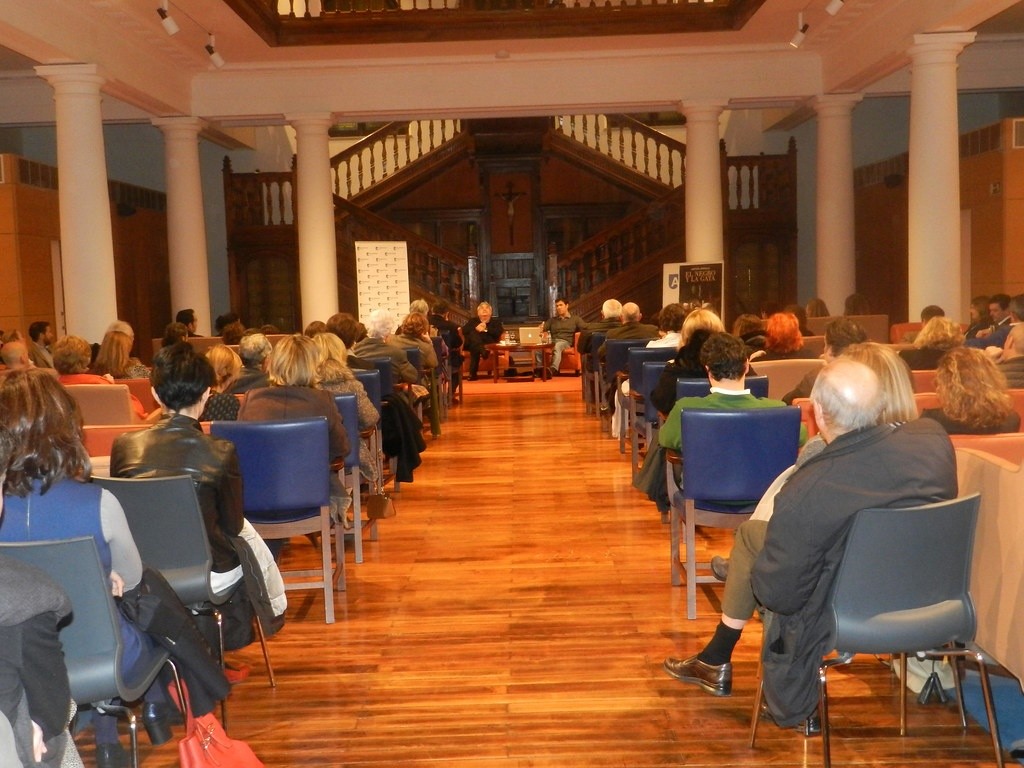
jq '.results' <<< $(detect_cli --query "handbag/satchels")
[398,381,433,409]
[366,493,396,519]
[165,678,268,768]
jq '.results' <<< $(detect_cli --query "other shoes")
[224,661,251,684]
[468,372,478,381]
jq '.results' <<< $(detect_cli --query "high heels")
[97,744,138,768]
[144,705,186,744]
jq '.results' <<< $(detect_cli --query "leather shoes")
[663,655,732,696]
[760,700,821,731]
[710,556,729,581]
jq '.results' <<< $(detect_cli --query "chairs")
[583,316,1024,768]
[0,335,463,768]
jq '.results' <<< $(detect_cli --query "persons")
[0,369,251,768]
[110,299,465,594]
[462,303,503,380]
[95,322,150,378]
[962,294,1024,359]
[0,329,32,369]
[0,423,85,768]
[539,298,585,379]
[53,336,113,383]
[29,321,54,367]
[576,299,964,734]
[921,348,1020,434]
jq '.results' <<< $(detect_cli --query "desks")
[484,342,555,383]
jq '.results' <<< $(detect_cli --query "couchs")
[542,332,581,377]
[458,327,498,376]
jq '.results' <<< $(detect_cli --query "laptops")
[520,327,541,343]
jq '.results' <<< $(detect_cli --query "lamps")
[156,0,180,36]
[790,12,809,48]
[825,0,845,16]
[205,33,225,69]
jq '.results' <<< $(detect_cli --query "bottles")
[505,331,510,346]
[547,331,552,344]
